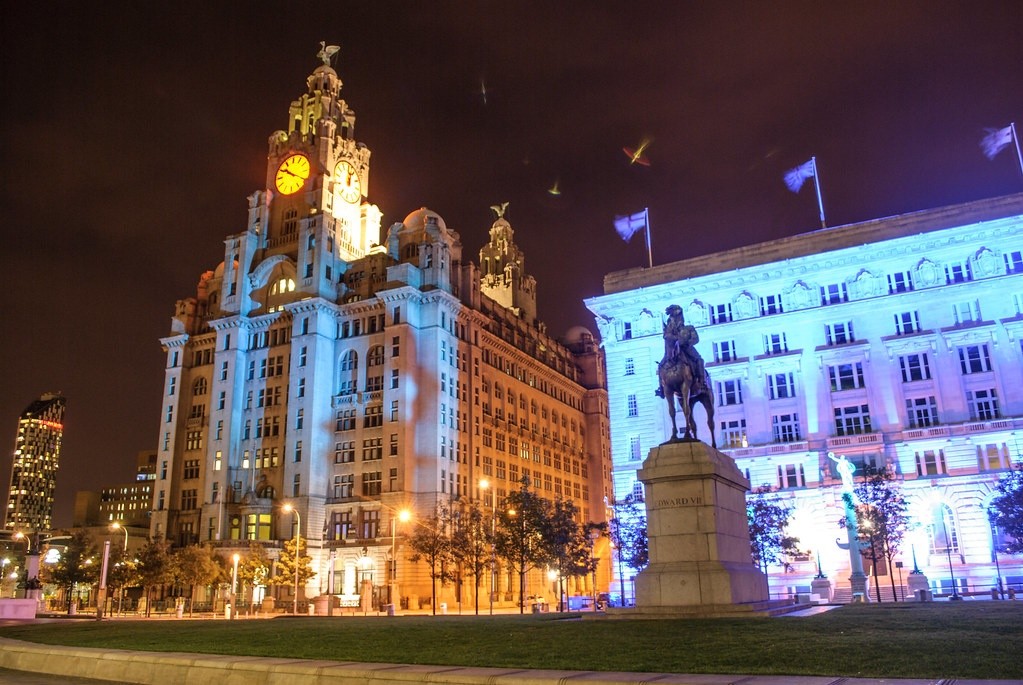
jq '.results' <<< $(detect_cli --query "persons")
[655,306,708,399]
[832,454,854,486]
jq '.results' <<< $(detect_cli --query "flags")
[613,209,645,244]
[783,159,815,194]
[979,125,1012,161]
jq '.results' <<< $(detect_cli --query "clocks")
[334,161,361,204]
[275,154,310,195]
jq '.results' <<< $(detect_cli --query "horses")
[658,320,717,448]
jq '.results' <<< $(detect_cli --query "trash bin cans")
[1007,587,1015,599]
[175,605,183,618]
[69,603,78,615]
[224,604,230,619]
[543,603,549,612]
[532,603,541,613]
[920,589,927,602]
[440,603,447,614]
[853,592,865,602]
[306,604,315,615]
[990,587,999,600]
[386,603,395,615]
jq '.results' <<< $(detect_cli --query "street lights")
[391,512,410,602]
[508,510,525,615]
[284,505,301,615]
[480,480,495,615]
[113,523,129,613]
[231,554,240,620]
